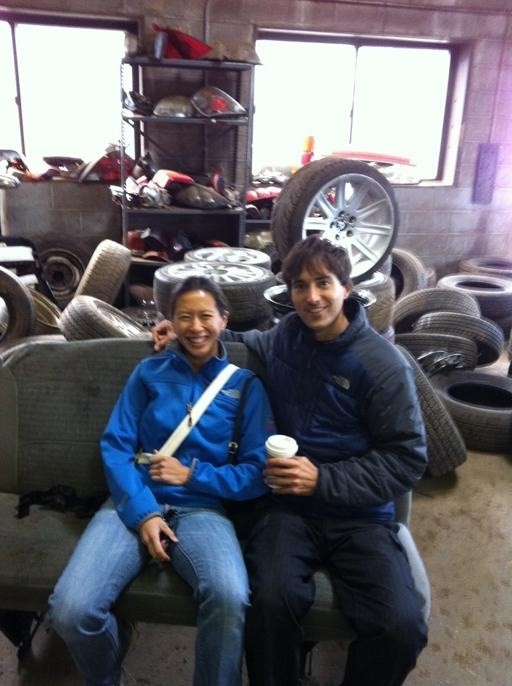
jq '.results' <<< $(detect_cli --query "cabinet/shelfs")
[120,55,254,310]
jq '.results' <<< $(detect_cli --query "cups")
[265,434,299,489]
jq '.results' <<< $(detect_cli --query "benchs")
[0,337,430,686]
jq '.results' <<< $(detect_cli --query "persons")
[44,276,276,686]
[153,236,429,686]
